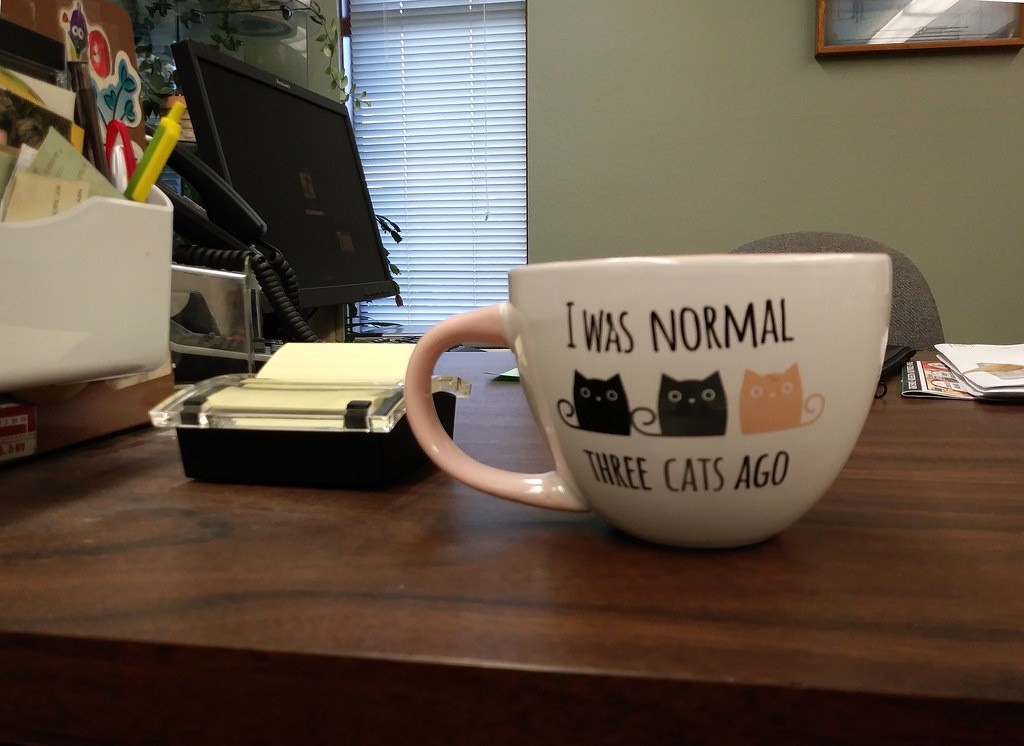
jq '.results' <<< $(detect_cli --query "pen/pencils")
[124,101,187,203]
[67,61,108,180]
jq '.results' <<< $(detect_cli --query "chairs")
[732,229,945,381]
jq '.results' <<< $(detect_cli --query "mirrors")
[814,0,1024,58]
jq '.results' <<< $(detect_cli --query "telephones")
[143,119,268,264]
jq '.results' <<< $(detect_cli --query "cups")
[404,253,892,547]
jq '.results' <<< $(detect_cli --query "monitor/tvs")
[170,38,398,314]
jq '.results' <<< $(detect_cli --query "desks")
[0,353,1024,746]
